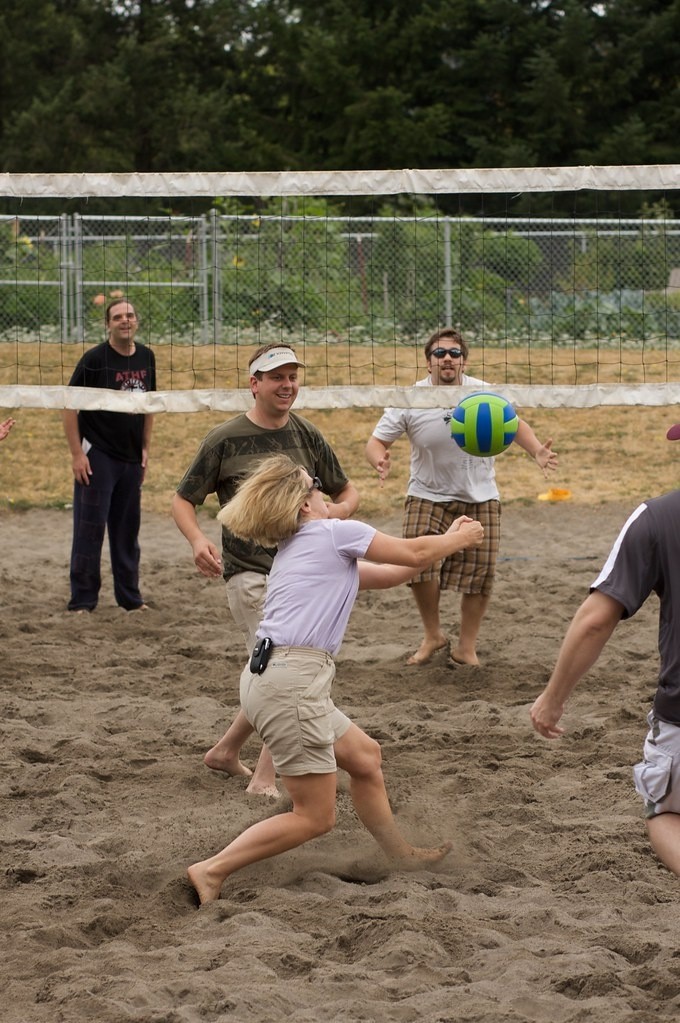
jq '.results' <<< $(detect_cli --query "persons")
[170,341,360,796]
[58,300,158,612]
[0,416,17,442]
[528,419,680,872]
[364,329,559,670]
[186,454,485,911]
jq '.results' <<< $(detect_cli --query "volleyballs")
[451,392,519,458]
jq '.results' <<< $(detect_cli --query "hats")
[249,347,307,376]
[667,423,680,440]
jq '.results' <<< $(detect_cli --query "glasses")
[308,477,323,492]
[428,347,464,358]
